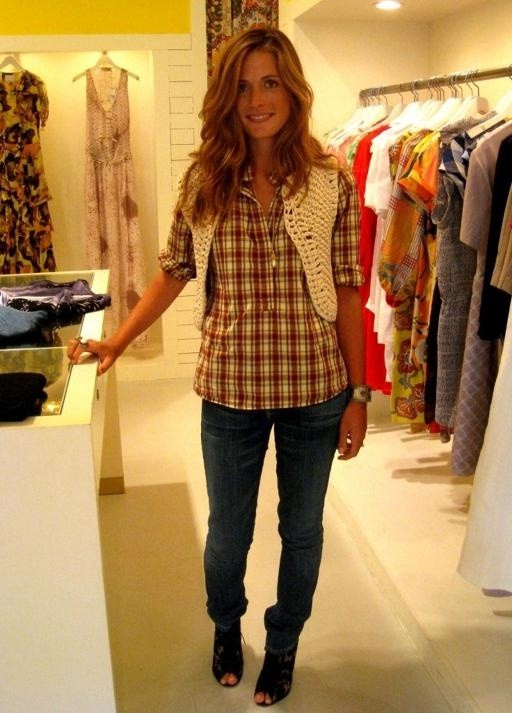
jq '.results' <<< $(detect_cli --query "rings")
[361,441,364,447]
[80,340,87,349]
[75,336,82,341]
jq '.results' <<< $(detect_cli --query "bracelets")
[348,384,372,402]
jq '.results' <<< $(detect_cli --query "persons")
[66,26,367,707]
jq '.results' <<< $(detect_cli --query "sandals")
[213,619,243,687]
[254,643,298,707]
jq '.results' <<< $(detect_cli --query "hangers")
[71,51,141,83]
[343,66,512,139]
[0,53,28,74]
